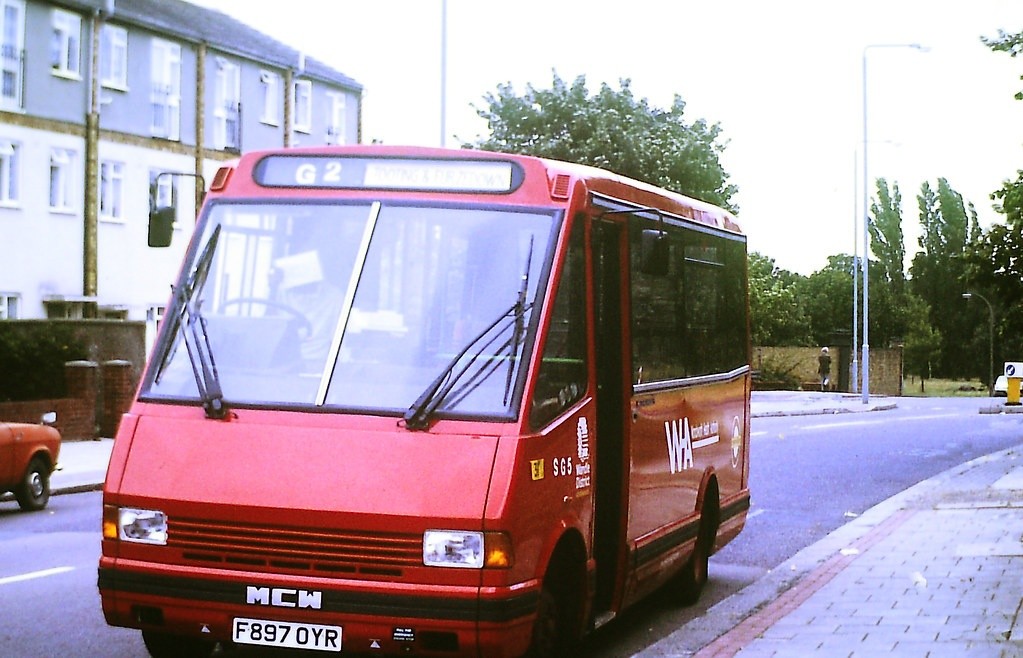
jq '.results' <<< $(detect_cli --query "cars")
[0,421,61,511]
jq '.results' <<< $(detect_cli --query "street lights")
[861,42,931,405]
[962,292,993,397]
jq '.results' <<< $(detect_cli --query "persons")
[818,347,832,391]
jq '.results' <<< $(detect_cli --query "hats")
[821,347,828,352]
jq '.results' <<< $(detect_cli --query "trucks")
[994,362,1022,398]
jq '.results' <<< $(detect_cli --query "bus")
[97,146,753,657]
